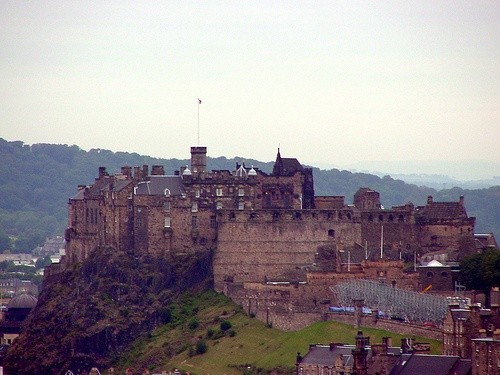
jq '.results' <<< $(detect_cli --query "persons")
[392,279,398,288]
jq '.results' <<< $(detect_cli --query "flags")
[199,100,201,103]
